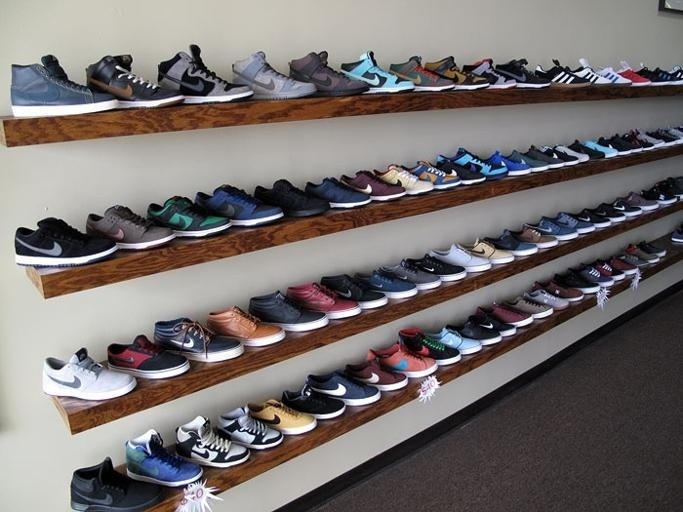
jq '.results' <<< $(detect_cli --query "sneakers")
[674,176,683,184]
[535,59,591,88]
[605,256,639,275]
[401,161,462,190]
[597,137,633,155]
[158,45,254,104]
[340,51,416,94]
[645,131,677,146]
[340,171,407,201]
[125,429,203,487]
[366,344,438,378]
[636,240,667,258]
[583,141,618,158]
[463,59,517,89]
[354,270,418,300]
[524,145,566,169]
[501,296,554,319]
[397,328,461,365]
[477,301,535,328]
[540,146,580,166]
[14,217,119,267]
[620,130,655,150]
[244,399,317,435]
[282,385,346,420]
[372,164,435,195]
[659,128,683,145]
[216,404,283,450]
[467,314,516,337]
[287,282,361,319]
[305,370,381,406]
[70,457,166,511]
[86,205,176,251]
[634,63,672,86]
[305,177,372,209]
[553,274,601,294]
[388,56,455,92]
[511,226,559,249]
[461,237,515,265]
[640,187,677,205]
[611,133,643,153]
[567,263,615,287]
[232,51,316,100]
[249,290,329,332]
[175,416,250,468]
[446,322,502,346]
[580,258,626,281]
[674,126,683,132]
[320,274,388,310]
[425,327,482,355]
[405,253,467,282]
[625,243,660,264]
[566,58,612,85]
[344,360,408,392]
[194,184,284,227]
[585,203,626,223]
[484,231,538,257]
[595,67,632,85]
[523,216,579,241]
[10,55,118,118]
[571,208,612,228]
[86,55,185,111]
[288,51,370,97]
[655,177,683,200]
[380,264,441,290]
[616,61,651,86]
[207,306,286,347]
[147,196,232,238]
[506,150,549,172]
[616,191,659,211]
[552,211,596,235]
[484,151,532,176]
[254,180,330,217]
[154,318,245,362]
[671,228,683,242]
[532,280,584,302]
[616,253,650,269]
[107,335,190,380]
[553,143,590,163]
[429,243,492,273]
[568,140,605,160]
[437,147,509,181]
[495,59,551,88]
[664,128,682,138]
[633,128,666,149]
[429,159,487,186]
[611,200,642,217]
[524,290,569,311]
[42,348,137,401]
[669,65,683,80]
[424,57,490,91]
